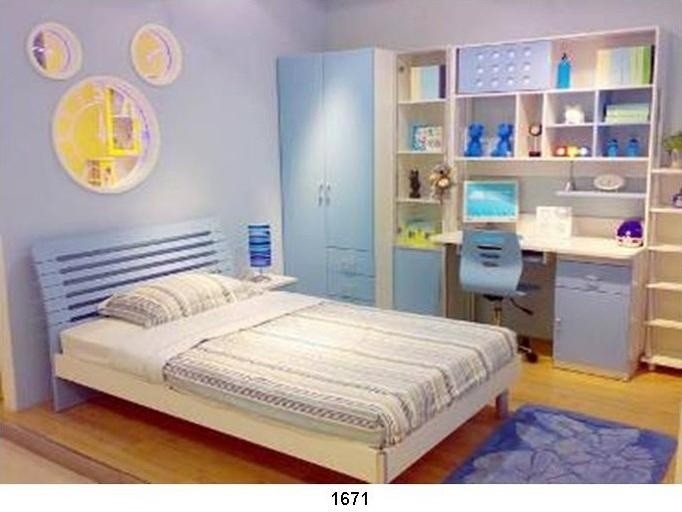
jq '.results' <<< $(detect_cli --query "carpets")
[440,404,678,483]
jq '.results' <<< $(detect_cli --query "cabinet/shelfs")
[277,26,681,382]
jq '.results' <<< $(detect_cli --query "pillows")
[98,271,265,328]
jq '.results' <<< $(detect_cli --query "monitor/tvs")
[462,180,519,222]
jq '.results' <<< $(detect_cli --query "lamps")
[249,225,271,281]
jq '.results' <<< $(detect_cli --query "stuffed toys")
[463,122,486,158]
[407,168,421,198]
[491,122,514,158]
[614,216,645,248]
[428,163,456,206]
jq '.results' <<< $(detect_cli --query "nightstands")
[252,273,299,292]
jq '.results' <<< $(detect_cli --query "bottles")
[557,53,570,88]
[607,138,640,157]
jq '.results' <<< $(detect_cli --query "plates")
[593,174,626,191]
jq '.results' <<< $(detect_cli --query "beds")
[31,219,523,483]
